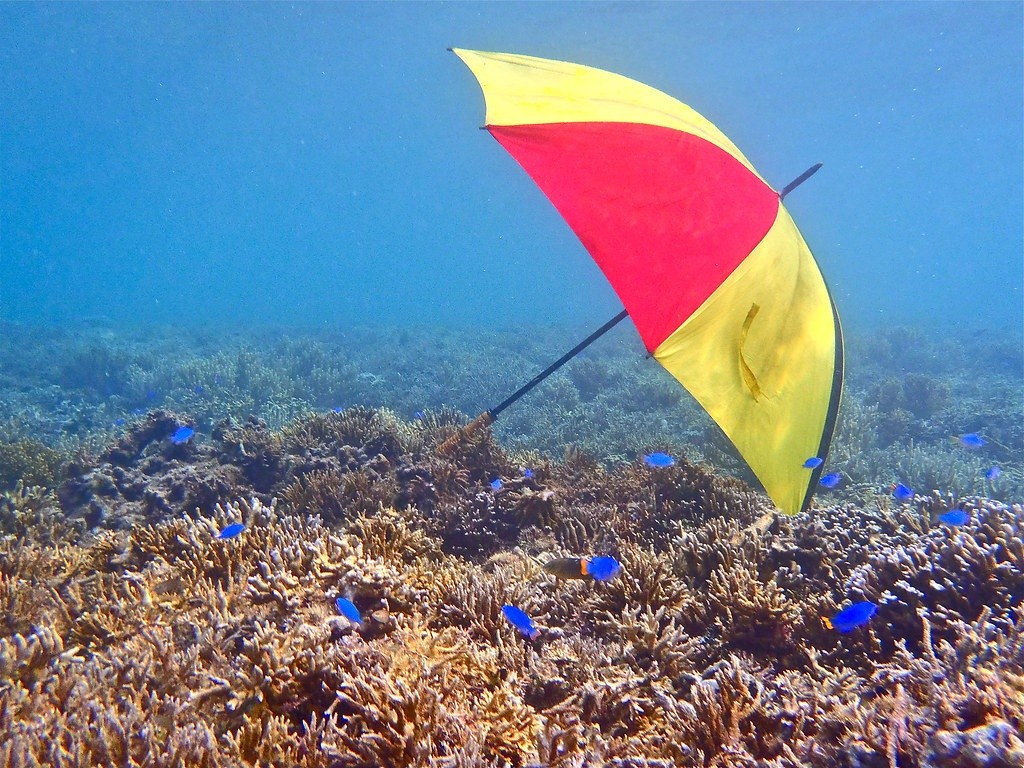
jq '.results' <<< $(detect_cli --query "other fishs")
[800,457,844,490]
[502,604,542,642]
[217,521,246,541]
[335,596,370,629]
[170,426,195,446]
[640,451,677,469]
[541,556,626,584]
[488,469,536,492]
[890,432,999,528]
[819,601,878,634]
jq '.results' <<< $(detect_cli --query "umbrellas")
[436,47,845,514]
